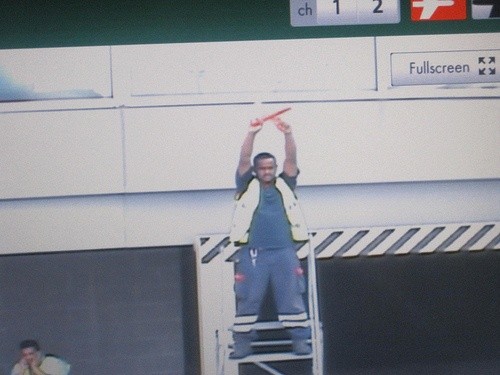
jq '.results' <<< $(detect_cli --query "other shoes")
[292,341,311,354]
[228,341,253,359]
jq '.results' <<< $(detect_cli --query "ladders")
[215,234,325,374]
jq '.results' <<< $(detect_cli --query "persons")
[9,339,70,375]
[226,110,318,360]
